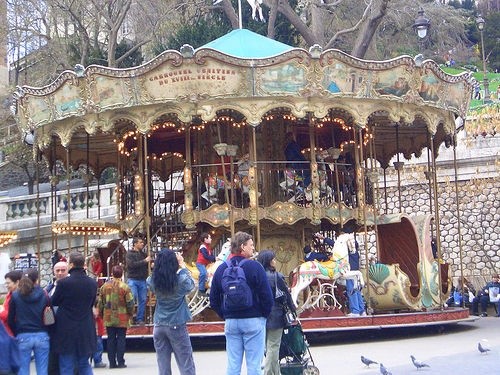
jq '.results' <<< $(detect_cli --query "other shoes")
[139,319,145,326]
[199,291,208,297]
[481,312,487,317]
[360,312,366,316]
[495,312,500,317]
[118,361,127,368]
[110,360,117,368]
[296,184,303,192]
[474,312,479,316]
[345,313,359,317]
[94,361,106,368]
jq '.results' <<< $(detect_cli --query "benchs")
[451,301,498,314]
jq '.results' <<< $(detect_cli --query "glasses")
[53,268,67,274]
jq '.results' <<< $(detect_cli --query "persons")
[284,131,311,194]
[196,231,221,297]
[209,231,310,375]
[126,236,152,326]
[443,273,500,317]
[303,245,330,262]
[0,248,135,375]
[237,152,250,176]
[315,230,367,317]
[145,248,195,375]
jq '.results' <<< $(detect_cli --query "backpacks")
[223,258,253,311]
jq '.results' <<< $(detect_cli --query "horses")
[280,146,333,196]
[186,238,233,298]
[288,232,364,308]
[201,153,249,203]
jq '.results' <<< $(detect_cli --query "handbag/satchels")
[275,271,287,305]
[42,288,55,326]
[349,252,360,270]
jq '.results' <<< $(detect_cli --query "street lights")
[411,7,432,60]
[475,14,493,104]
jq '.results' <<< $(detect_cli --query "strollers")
[262,312,320,375]
